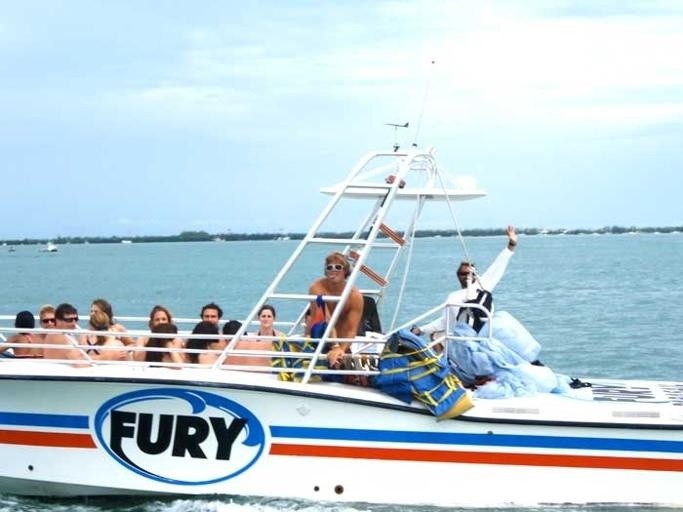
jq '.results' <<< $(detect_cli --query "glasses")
[325,264,344,271]
[60,317,78,322]
[40,318,56,323]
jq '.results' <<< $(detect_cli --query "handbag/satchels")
[311,294,330,354]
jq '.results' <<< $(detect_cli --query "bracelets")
[509,240,516,246]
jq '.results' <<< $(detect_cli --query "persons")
[442,289,543,387]
[308,252,365,385]
[2,296,382,376]
[408,223,518,356]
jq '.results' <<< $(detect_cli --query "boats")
[38,239,58,253]
[0,115,683,512]
